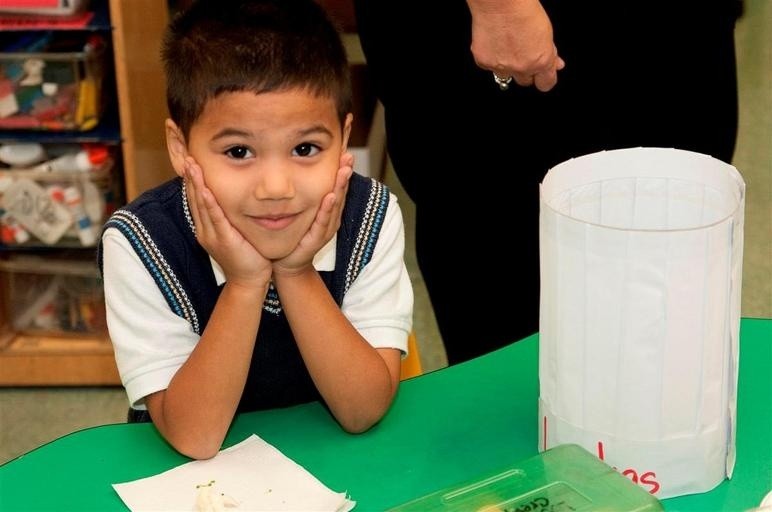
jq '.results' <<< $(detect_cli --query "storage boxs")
[391,445,665,512]
[0,258,111,340]
[1,157,119,251]
[1,48,104,134]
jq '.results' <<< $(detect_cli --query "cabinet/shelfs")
[0,0,178,390]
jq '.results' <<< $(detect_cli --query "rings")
[491,70,514,90]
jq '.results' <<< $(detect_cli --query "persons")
[101,0,416,460]
[354,0,747,369]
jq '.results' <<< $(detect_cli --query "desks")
[1,309,772,512]
